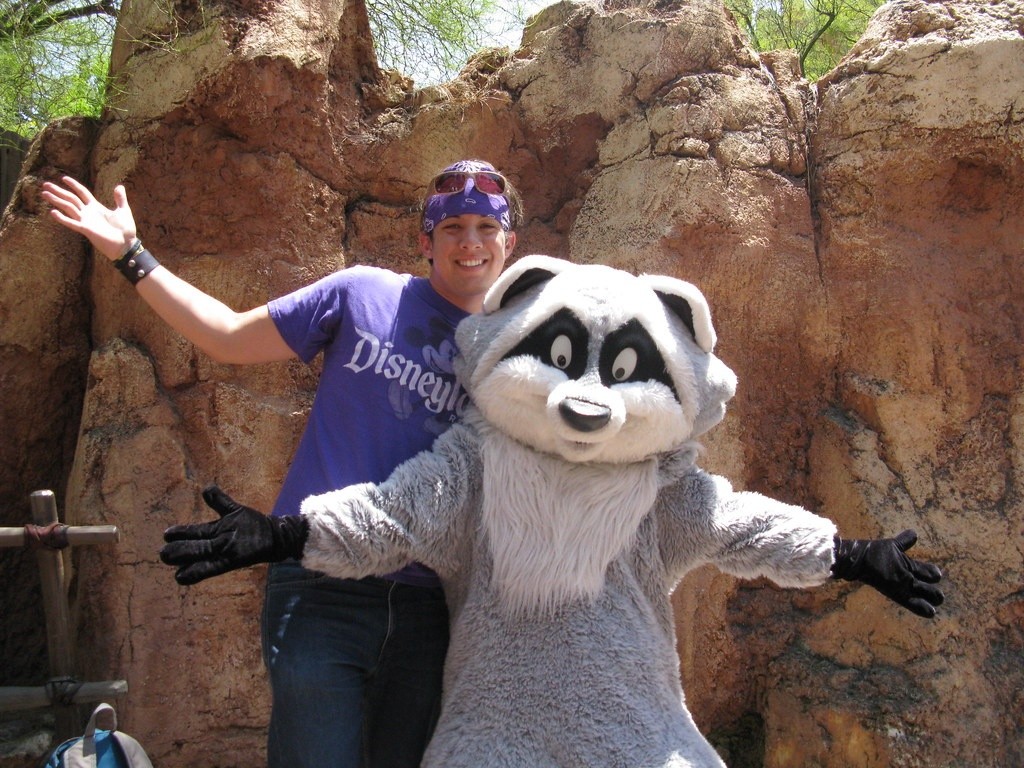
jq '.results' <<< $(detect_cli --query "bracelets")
[113,239,160,285]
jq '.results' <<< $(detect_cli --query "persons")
[42,160,525,768]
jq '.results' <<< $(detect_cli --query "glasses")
[419,171,518,232]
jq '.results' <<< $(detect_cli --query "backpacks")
[36,703,154,768]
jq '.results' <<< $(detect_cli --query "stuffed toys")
[160,255,944,768]
